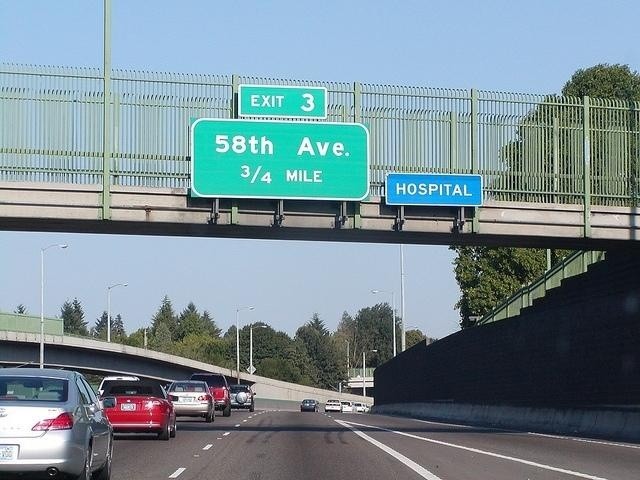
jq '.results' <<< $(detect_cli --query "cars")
[0,368,256,480]
[301,399,374,413]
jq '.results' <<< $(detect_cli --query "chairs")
[175,387,183,392]
[195,387,203,392]
[37,392,60,400]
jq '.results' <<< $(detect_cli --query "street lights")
[107,283,128,343]
[362,350,377,397]
[40,243,68,369]
[237,306,267,385]
[371,290,396,358]
